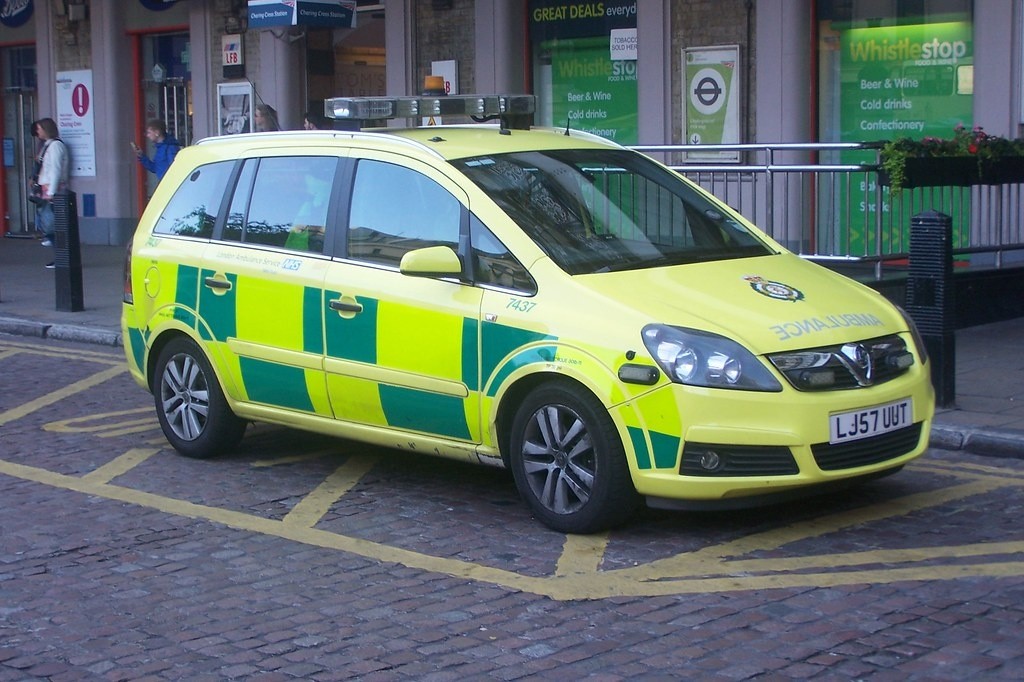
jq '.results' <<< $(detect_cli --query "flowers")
[876,123,1024,197]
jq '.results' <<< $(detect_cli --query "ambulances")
[121,89,938,536]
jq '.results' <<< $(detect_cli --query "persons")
[255,104,279,132]
[303,112,323,130]
[219,94,250,134]
[130,120,185,182]
[29,118,70,248]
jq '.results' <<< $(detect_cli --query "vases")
[879,155,1023,189]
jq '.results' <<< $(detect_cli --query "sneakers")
[41,240,53,247]
[45,262,55,269]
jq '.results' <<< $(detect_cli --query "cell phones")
[130,142,139,150]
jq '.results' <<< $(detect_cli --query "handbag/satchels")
[28,183,42,204]
